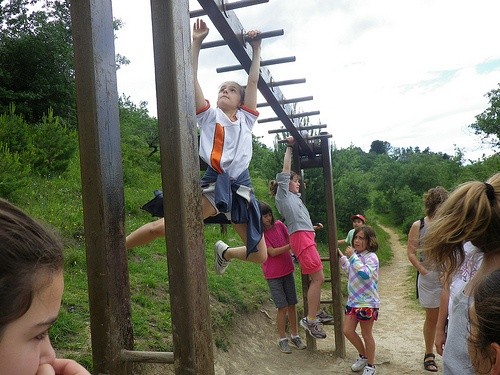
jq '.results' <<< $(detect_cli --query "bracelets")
[285,143,294,148]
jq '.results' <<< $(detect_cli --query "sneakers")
[213,238,232,277]
[315,308,334,323]
[350,353,368,372]
[277,336,292,353]
[361,362,377,375]
[289,333,307,351]
[298,316,328,339]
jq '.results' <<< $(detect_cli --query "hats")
[350,213,366,224]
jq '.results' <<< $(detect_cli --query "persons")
[337,214,367,246]
[126,16,269,275]
[407,172,500,375]
[337,225,381,375]
[273,135,328,340]
[256,202,324,354]
[0,197,91,375]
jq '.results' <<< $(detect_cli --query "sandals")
[423,352,439,372]
